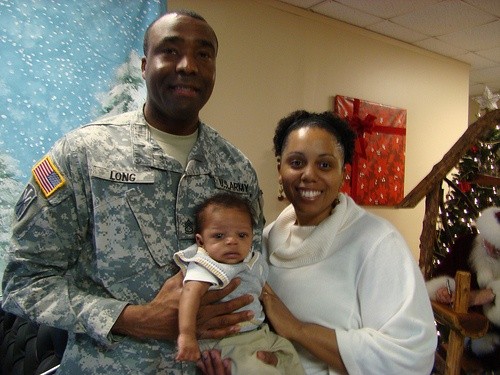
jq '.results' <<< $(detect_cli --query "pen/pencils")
[446,278,452,296]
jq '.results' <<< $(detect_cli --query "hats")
[476,207,500,250]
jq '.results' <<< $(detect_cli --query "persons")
[172,193,308,375]
[2,10,264,375]
[261,110,438,375]
